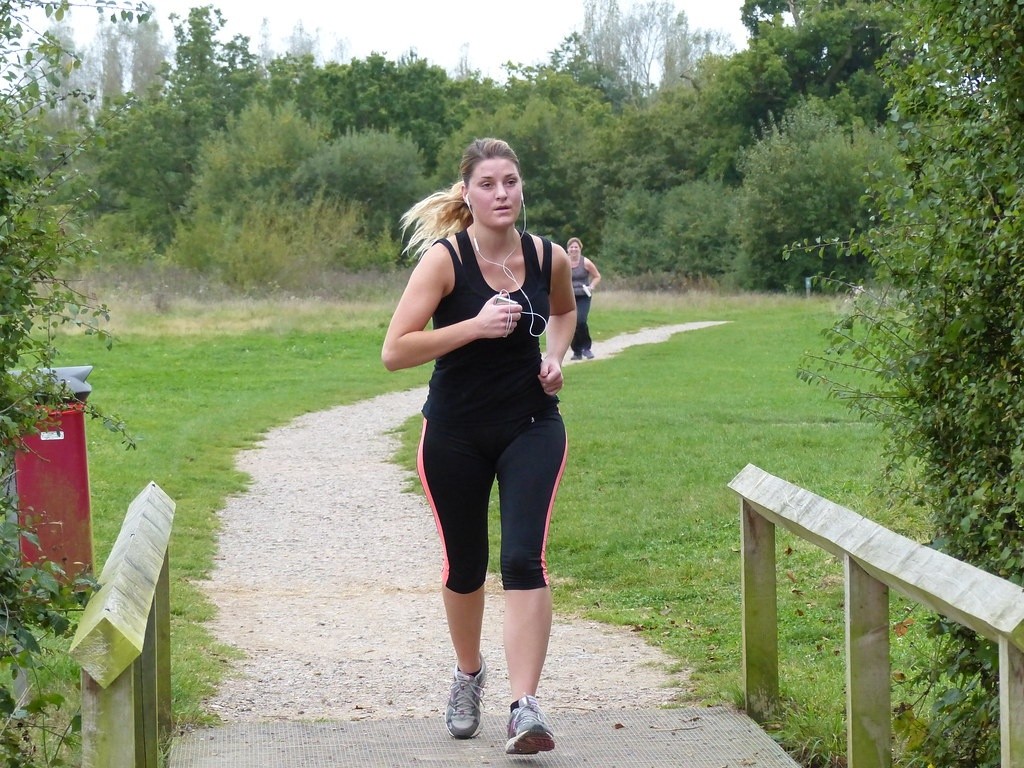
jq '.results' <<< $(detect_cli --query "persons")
[380,138,577,754]
[567,238,601,360]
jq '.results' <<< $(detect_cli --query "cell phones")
[494,296,518,306]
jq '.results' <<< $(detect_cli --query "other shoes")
[570,354,582,360]
[582,348,594,359]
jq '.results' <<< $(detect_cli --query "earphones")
[521,193,524,201]
[466,193,470,208]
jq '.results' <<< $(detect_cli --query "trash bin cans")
[6,365,96,593]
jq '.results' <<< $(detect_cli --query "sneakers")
[506,692,555,755]
[445,652,486,740]
